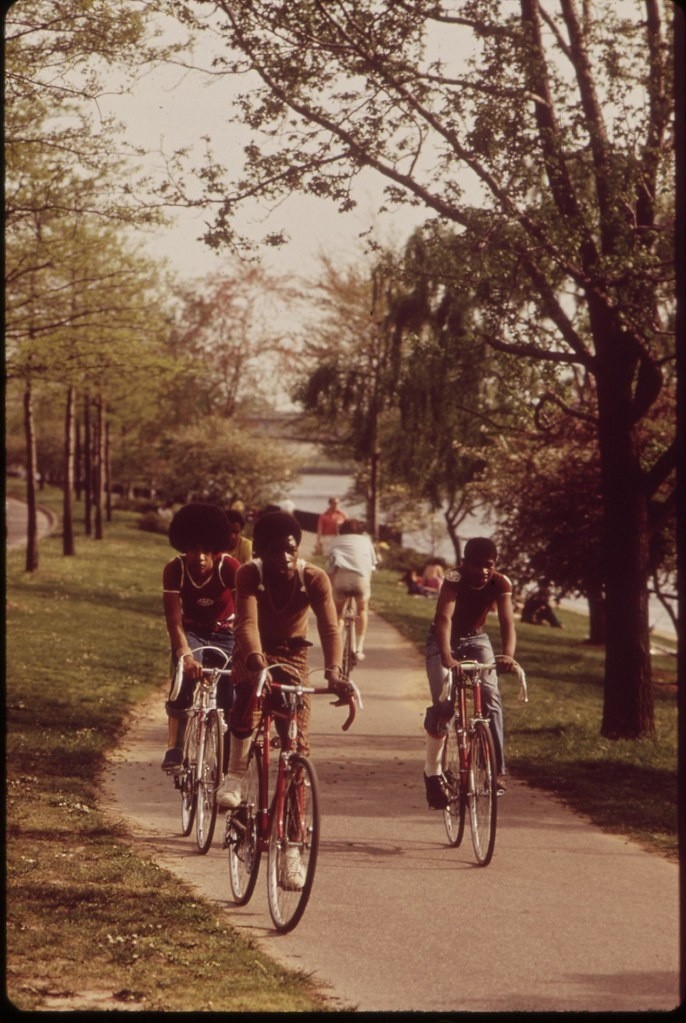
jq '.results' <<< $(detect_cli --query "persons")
[222,508,253,566]
[422,536,518,808]
[522,587,564,628]
[213,515,350,891]
[407,559,447,595]
[321,517,378,662]
[317,496,348,552]
[160,504,247,810]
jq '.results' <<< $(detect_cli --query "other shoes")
[355,652,365,660]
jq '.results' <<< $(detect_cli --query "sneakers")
[482,779,507,796]
[161,747,183,771]
[216,775,243,807]
[280,845,307,888]
[423,771,452,810]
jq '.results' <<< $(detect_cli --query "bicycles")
[436,656,531,867]
[220,662,365,934]
[163,643,241,858]
[335,589,362,684]
